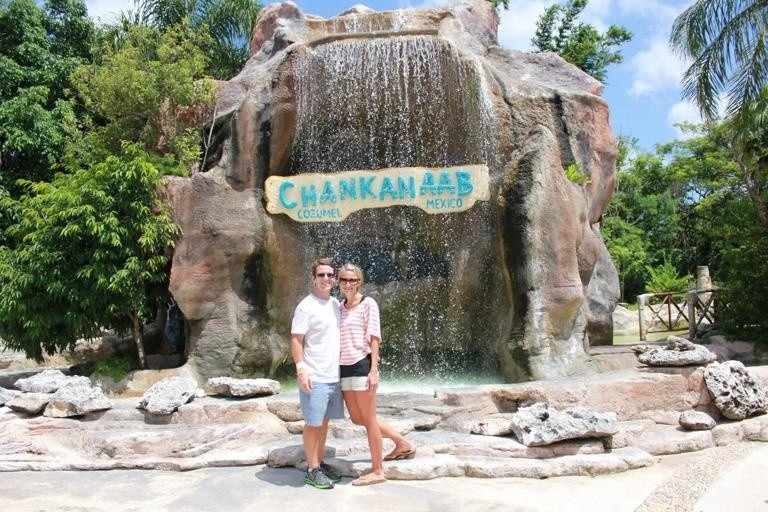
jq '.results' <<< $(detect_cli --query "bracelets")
[294,361,308,377]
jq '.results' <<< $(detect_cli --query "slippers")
[352,474,387,486]
[384,448,416,461]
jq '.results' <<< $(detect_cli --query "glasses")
[317,273,335,278]
[340,278,360,286]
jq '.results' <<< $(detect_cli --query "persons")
[289,258,345,490]
[338,264,416,486]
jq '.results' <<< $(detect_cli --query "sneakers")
[304,461,341,489]
